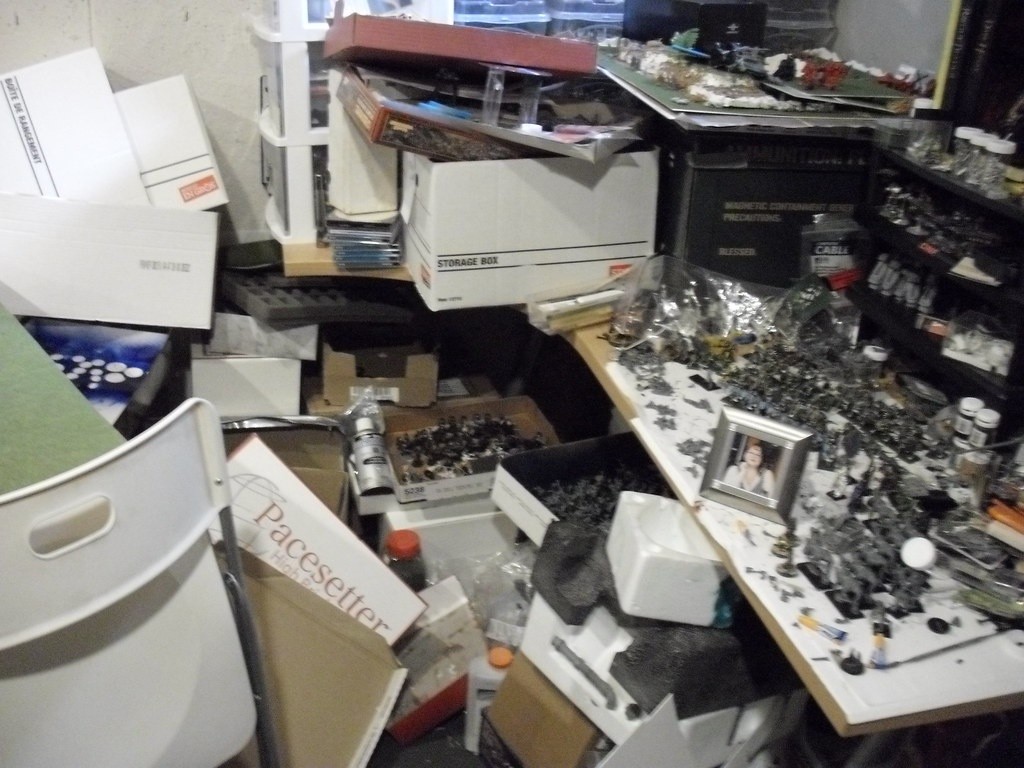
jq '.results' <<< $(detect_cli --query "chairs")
[0,396,280,768]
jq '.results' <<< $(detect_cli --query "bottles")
[906,108,952,165]
[965,133,1000,183]
[382,528,425,593]
[465,647,512,755]
[352,417,394,496]
[953,127,984,177]
[981,140,1016,199]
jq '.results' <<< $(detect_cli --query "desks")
[274,237,1024,738]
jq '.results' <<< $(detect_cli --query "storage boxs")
[0,12,660,768]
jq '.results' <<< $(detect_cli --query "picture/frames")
[700,402,815,524]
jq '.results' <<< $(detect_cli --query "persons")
[723,444,775,498]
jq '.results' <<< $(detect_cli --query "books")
[0,47,229,211]
[321,208,403,270]
[209,434,428,647]
